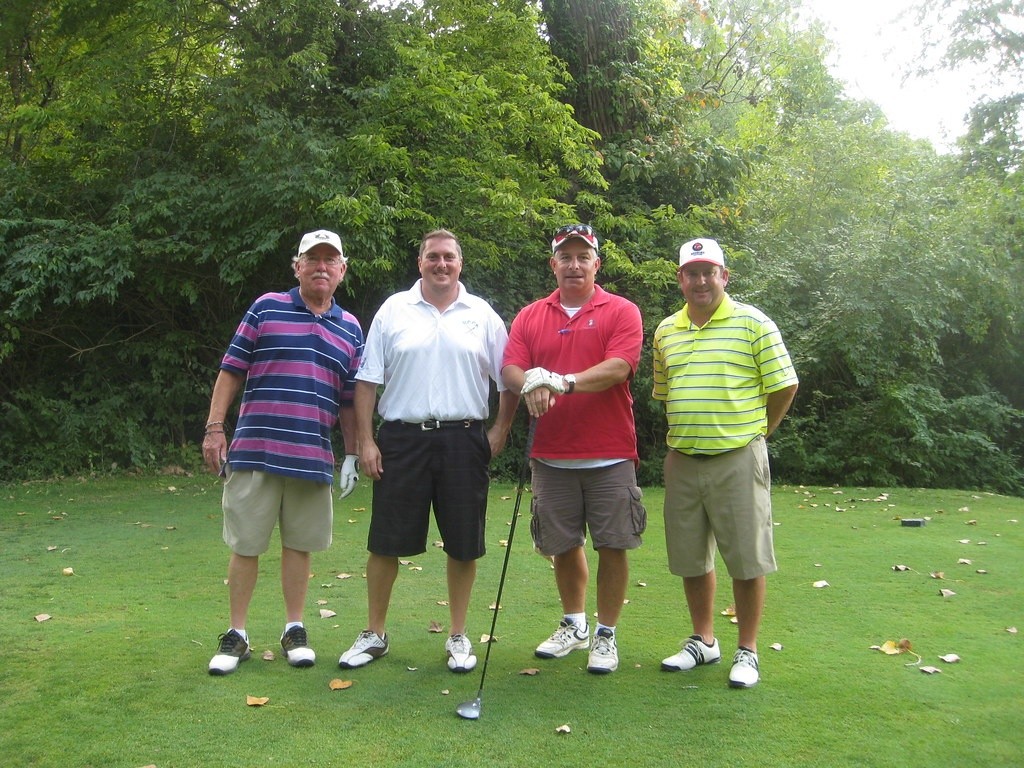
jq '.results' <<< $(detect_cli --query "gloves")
[339,454,360,499]
[520,367,565,396]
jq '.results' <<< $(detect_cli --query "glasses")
[299,252,346,266]
[554,225,596,238]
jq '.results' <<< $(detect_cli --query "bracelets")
[205,420,224,428]
[205,430,224,434]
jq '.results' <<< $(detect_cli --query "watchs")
[564,373,576,395]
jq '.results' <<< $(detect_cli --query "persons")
[337,228,521,675]
[652,237,799,688]
[500,222,647,673]
[203,229,366,677]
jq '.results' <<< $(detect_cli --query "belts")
[393,418,479,431]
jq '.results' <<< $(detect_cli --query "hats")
[298,229,343,258]
[679,238,725,270]
[551,228,598,257]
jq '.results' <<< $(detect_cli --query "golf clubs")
[454,414,539,721]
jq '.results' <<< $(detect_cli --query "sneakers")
[445,630,478,671]
[339,629,390,669]
[728,644,759,687]
[535,617,591,659]
[281,624,317,666]
[208,629,251,676]
[586,626,619,672]
[661,634,720,671]
